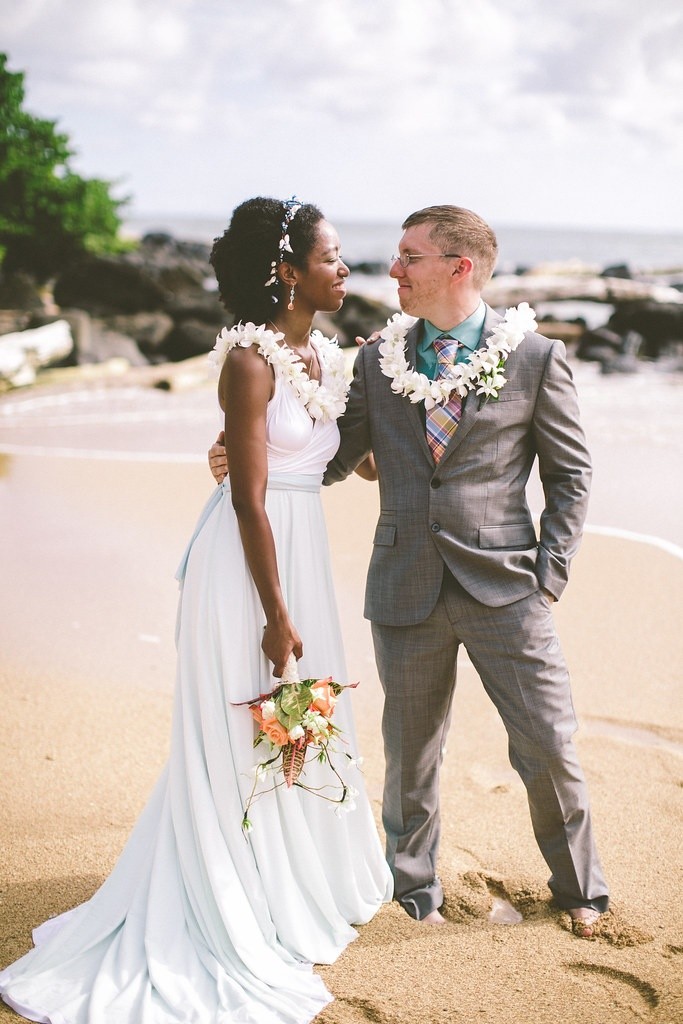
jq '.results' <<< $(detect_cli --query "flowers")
[229,651,365,842]
[473,359,508,399]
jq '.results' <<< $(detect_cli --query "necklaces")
[377,302,538,409]
[268,318,313,381]
[209,320,351,422]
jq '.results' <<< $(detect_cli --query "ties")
[425,338,464,468]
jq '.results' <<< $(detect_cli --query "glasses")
[390,254,462,268]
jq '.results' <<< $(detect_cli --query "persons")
[0,197,376,1024]
[208,205,611,925]
